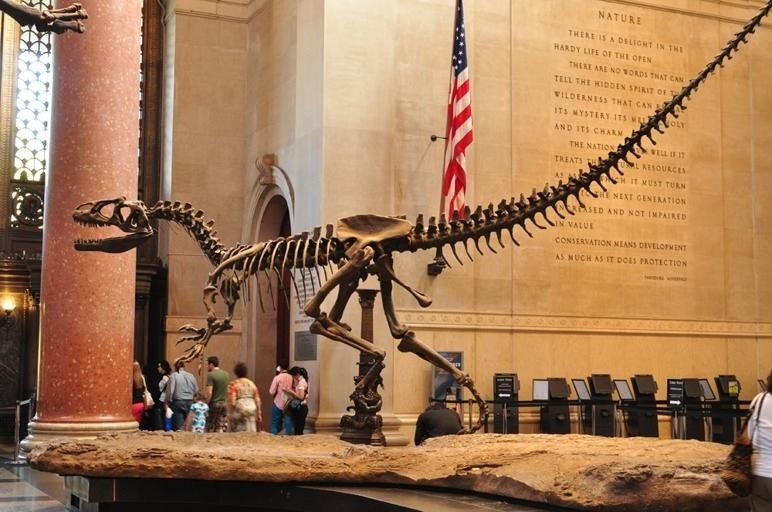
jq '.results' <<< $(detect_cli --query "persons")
[204,356,231,433]
[413,397,464,446]
[156,360,172,430]
[745,369,771,512]
[226,363,262,433]
[184,393,211,434]
[133,360,145,423]
[269,362,294,436]
[163,361,199,431]
[282,366,309,435]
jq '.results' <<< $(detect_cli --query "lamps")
[0,299,16,342]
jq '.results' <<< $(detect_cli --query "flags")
[441,0,473,222]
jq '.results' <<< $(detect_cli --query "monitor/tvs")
[547,377,570,398]
[682,378,702,397]
[719,374,736,394]
[592,374,614,394]
[635,374,657,394]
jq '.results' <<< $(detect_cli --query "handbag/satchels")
[285,398,303,417]
[720,431,755,499]
